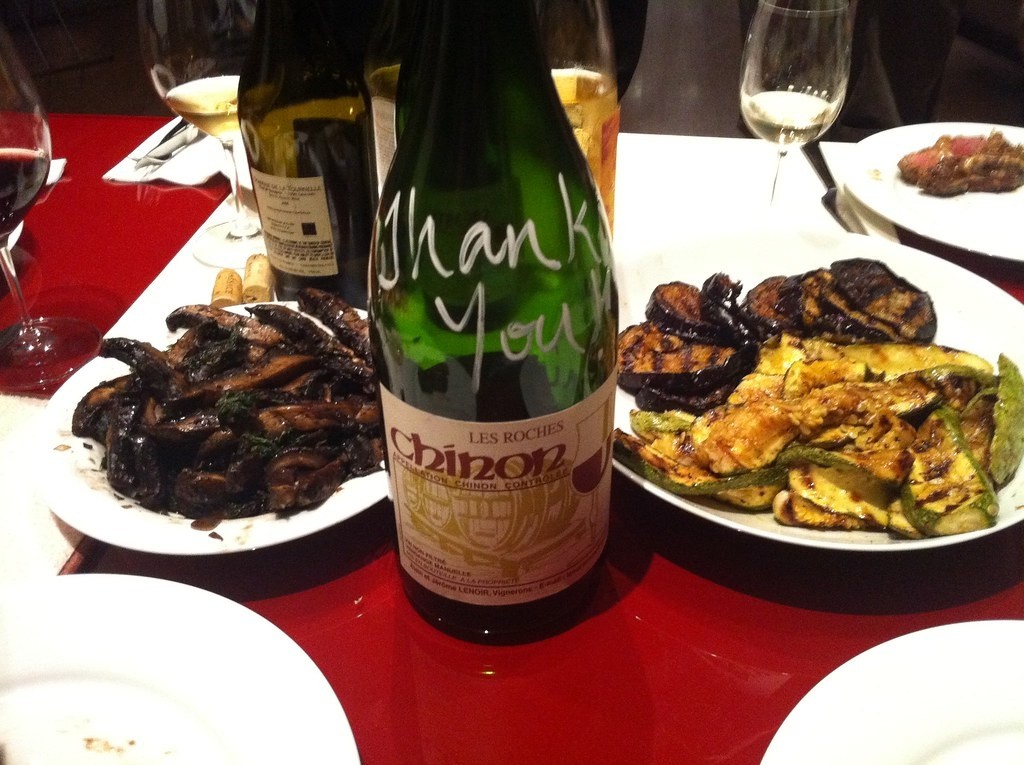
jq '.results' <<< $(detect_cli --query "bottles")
[537,0,619,244]
[364,0,410,208]
[238,0,379,310]
[365,2,619,644]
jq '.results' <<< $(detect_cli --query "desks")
[0,96,1024,765]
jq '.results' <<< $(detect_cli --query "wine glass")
[0,23,103,389]
[136,0,269,271]
[739,0,853,203]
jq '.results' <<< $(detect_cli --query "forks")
[125,116,191,166]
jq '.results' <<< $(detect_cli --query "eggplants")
[618,256,936,417]
[71,287,380,513]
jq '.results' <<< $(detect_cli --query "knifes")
[799,143,867,236]
[133,124,199,171]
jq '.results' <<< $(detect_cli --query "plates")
[612,230,1024,552]
[0,573,362,765]
[31,301,389,555]
[759,620,1024,765]
[838,121,1024,263]
